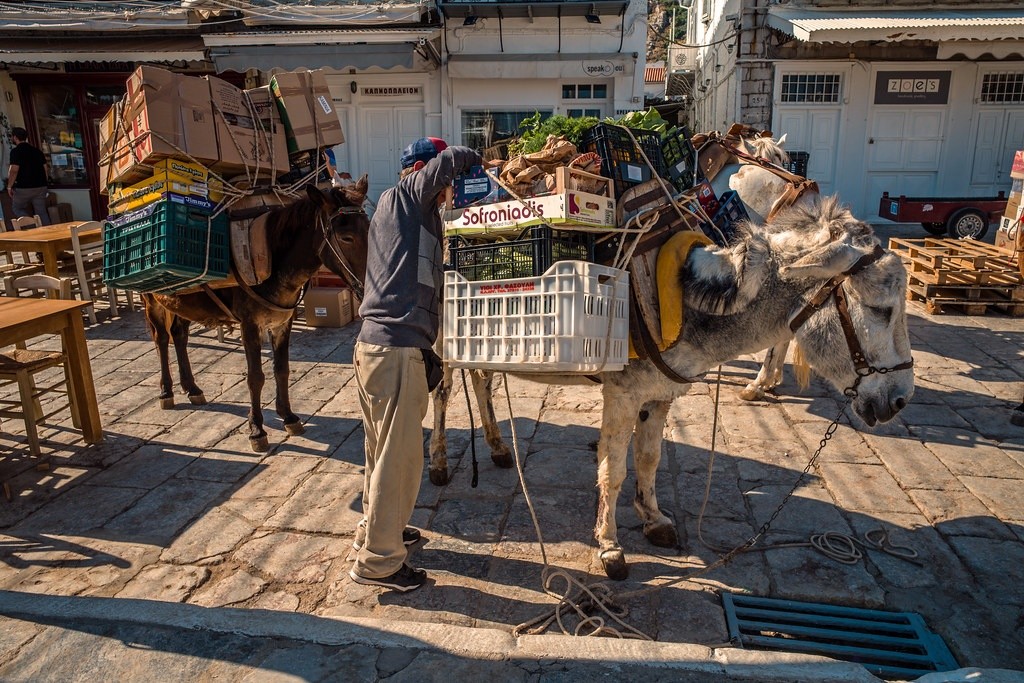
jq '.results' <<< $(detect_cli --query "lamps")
[463,12,478,25]
[585,9,601,24]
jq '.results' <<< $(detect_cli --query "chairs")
[0,214,135,455]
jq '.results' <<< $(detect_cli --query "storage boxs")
[775,150,810,180]
[995,150,1024,251]
[303,270,361,327]
[440,123,750,378]
[99,65,346,295]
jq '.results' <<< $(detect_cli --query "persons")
[7,127,50,230]
[346,137,504,591]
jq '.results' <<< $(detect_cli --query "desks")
[0,296,103,445]
[0,221,101,299]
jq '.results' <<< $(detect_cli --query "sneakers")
[353,526,421,551]
[350,562,426,593]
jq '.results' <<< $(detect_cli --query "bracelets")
[7,188,11,189]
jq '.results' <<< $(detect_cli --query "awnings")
[767,5,1024,45]
[0,41,208,62]
[201,28,442,47]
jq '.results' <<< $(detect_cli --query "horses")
[139,173,369,449]
[424,131,916,581]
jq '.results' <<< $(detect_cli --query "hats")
[400,137,449,170]
